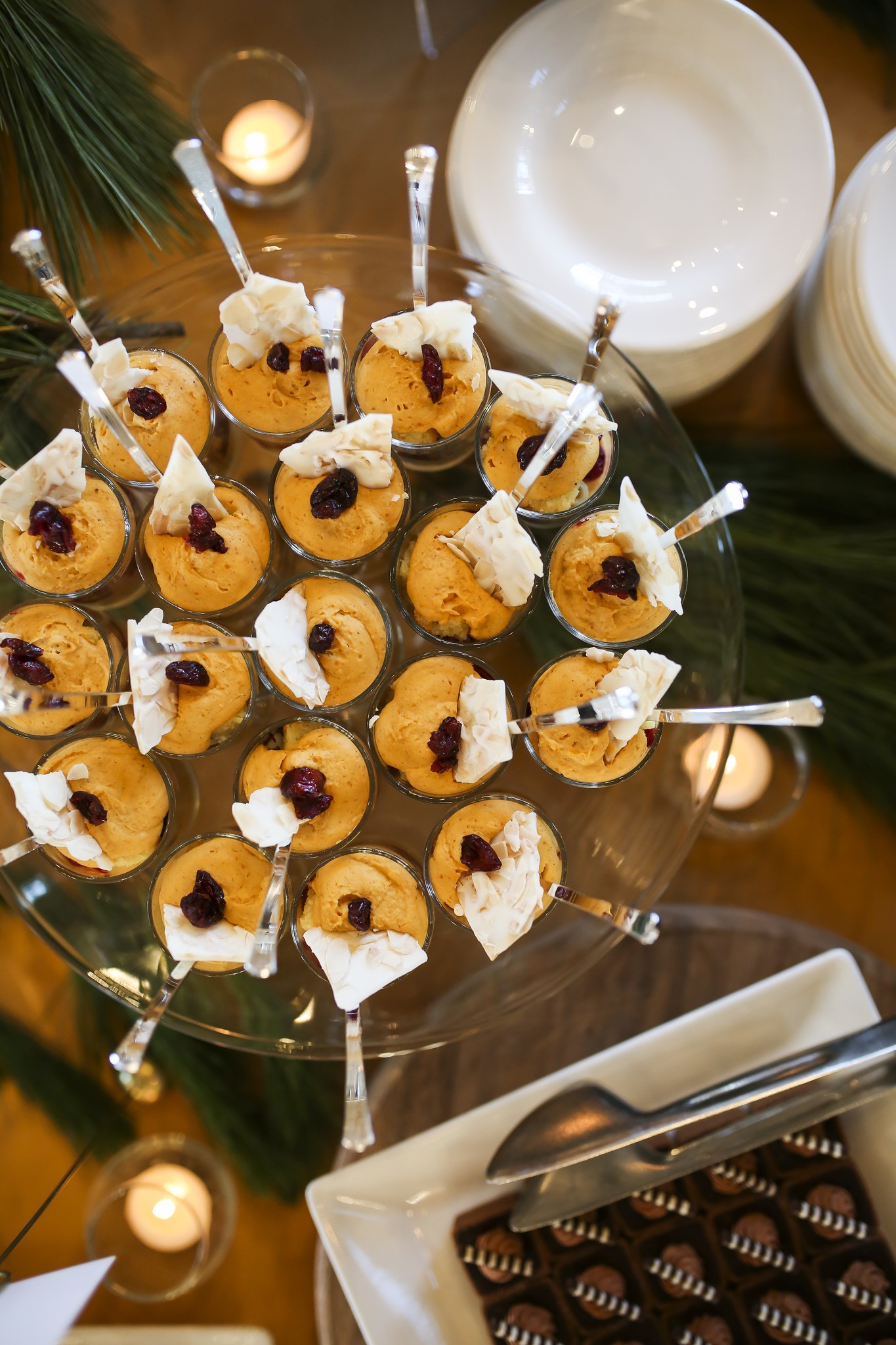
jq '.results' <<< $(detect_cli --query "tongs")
[484,1012,896,1235]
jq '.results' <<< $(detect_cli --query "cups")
[661,686,809,845]
[84,1135,238,1306]
[189,47,318,186]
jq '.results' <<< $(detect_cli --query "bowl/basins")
[0,221,752,1066]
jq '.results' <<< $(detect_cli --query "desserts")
[451,1113,895,1344]
[0,271,688,1013]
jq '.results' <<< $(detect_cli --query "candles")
[124,1163,215,1253]
[678,722,774,813]
[221,101,313,186]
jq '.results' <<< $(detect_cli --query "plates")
[430,2,896,485]
[300,941,896,1345]
